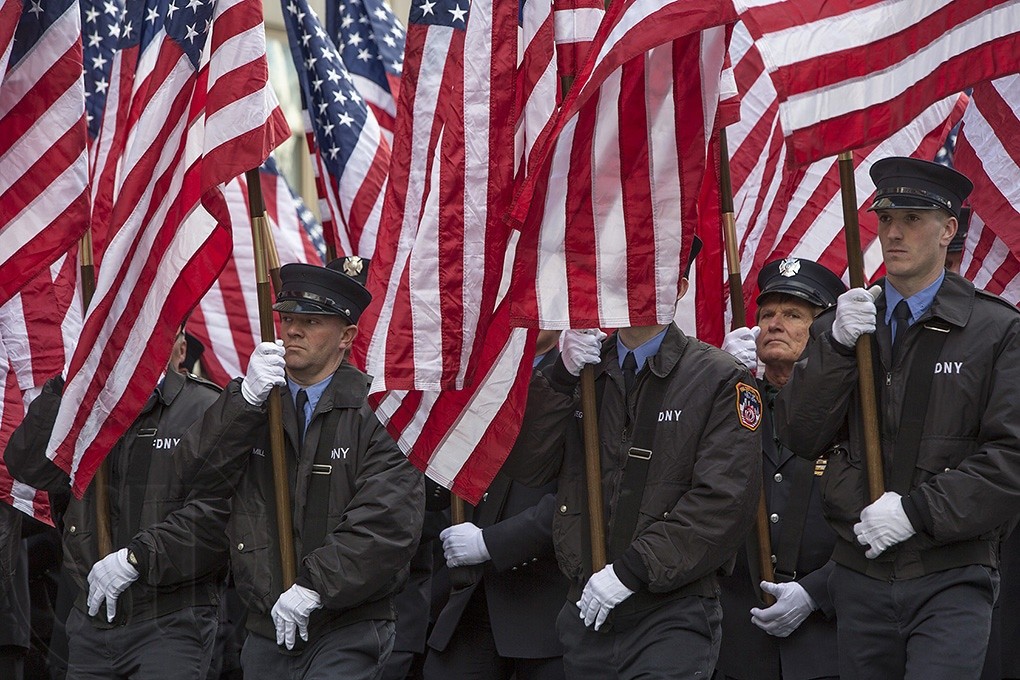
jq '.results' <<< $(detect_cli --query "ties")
[296,390,308,455]
[890,299,912,369]
[621,353,638,396]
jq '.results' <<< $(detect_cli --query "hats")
[269,261,372,325]
[181,330,205,373]
[326,257,372,287]
[946,206,972,253]
[681,233,702,279]
[755,257,848,310]
[866,157,975,212]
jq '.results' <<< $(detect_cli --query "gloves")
[720,326,761,370]
[86,547,138,621]
[241,339,286,406]
[853,491,915,559]
[832,285,881,348]
[61,363,70,381]
[440,522,490,569]
[574,562,636,631]
[750,580,816,638]
[271,582,323,649]
[560,328,608,377]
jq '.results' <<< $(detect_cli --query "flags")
[0,0,1020,524]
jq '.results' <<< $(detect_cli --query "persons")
[0,155,1020,680]
